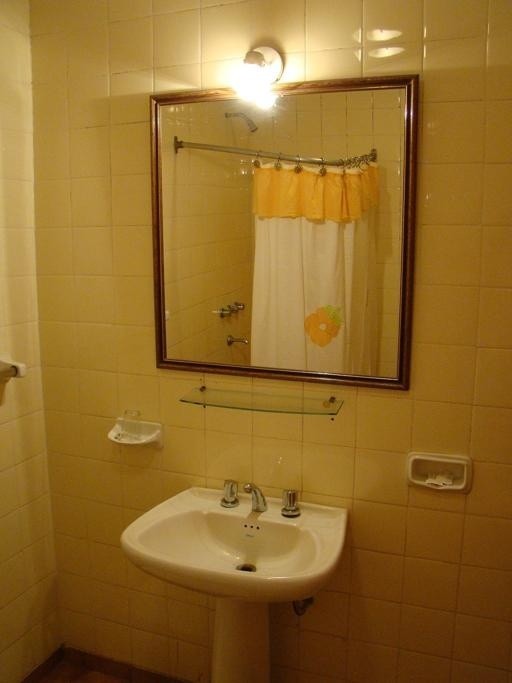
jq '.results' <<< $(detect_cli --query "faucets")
[243,481,267,512]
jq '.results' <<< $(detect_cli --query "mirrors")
[151,71,422,390]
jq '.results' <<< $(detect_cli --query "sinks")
[120,486,348,604]
